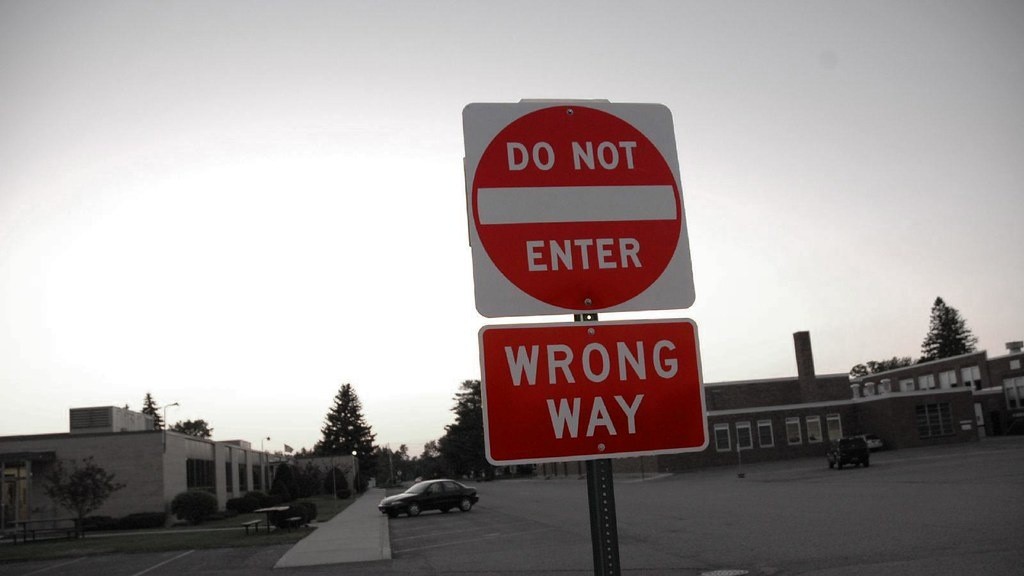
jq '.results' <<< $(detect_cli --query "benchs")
[243,520,260,534]
[288,516,301,528]
[2,525,85,543]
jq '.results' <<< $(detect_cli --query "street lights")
[332,454,341,502]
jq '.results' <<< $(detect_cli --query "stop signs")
[461,101,695,318]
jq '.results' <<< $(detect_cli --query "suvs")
[826,435,869,469]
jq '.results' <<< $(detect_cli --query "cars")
[415,476,423,482]
[476,471,490,482]
[864,434,883,450]
[377,480,479,518]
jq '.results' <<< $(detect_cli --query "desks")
[7,517,80,543]
[255,506,289,532]
